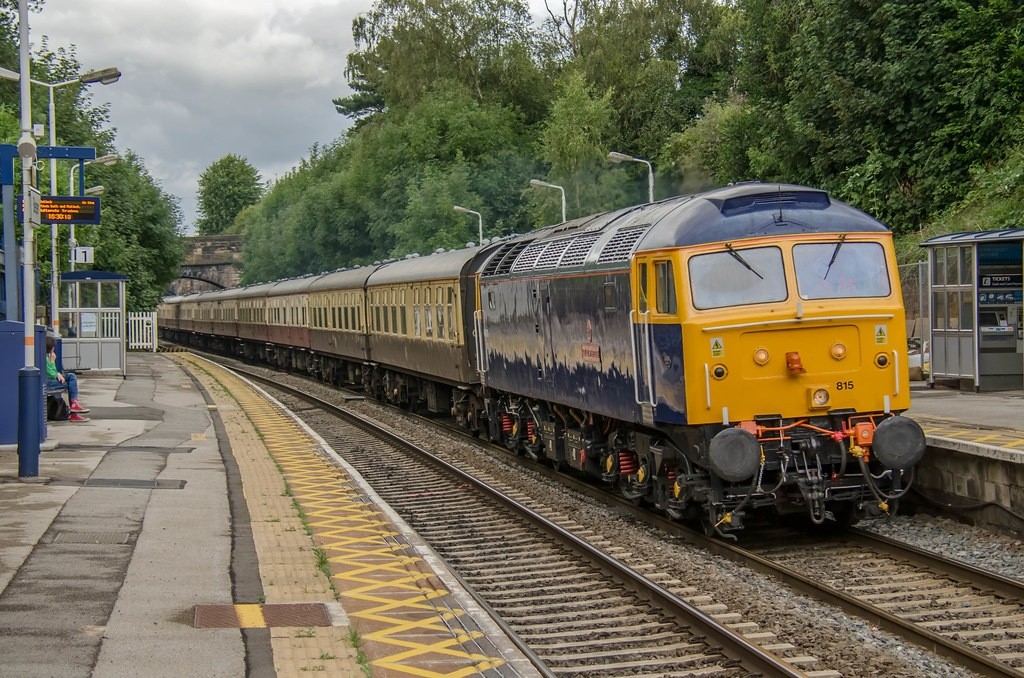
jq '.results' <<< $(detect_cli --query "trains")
[152,182,927,541]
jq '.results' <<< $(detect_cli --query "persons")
[45,337,91,422]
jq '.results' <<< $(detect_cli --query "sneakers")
[69,400,91,412]
[70,414,90,422]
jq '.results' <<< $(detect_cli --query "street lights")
[529,179,567,223]
[453,205,484,246]
[609,151,654,203]
[68,154,118,328]
[0,67,122,374]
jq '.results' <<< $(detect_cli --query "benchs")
[46,388,68,420]
